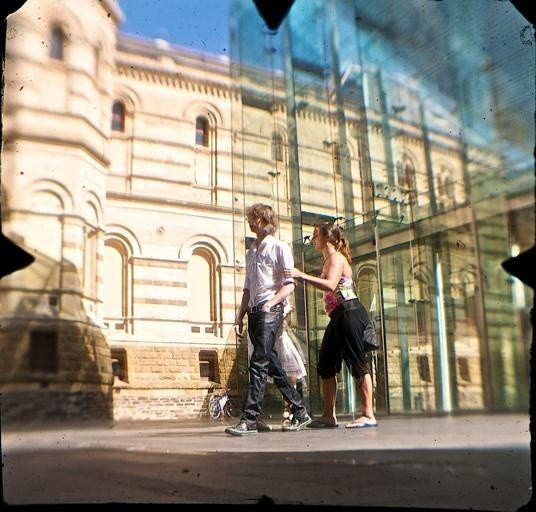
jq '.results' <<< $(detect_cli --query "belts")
[246,304,281,314]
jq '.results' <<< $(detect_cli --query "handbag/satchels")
[362,320,379,351]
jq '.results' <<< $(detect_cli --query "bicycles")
[206,385,237,420]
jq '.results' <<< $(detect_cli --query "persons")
[225,204,313,436]
[283,219,379,428]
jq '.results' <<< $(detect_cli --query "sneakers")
[282,414,312,431]
[225,418,259,435]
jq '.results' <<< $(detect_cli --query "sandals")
[306,420,338,427]
[345,420,378,428]
